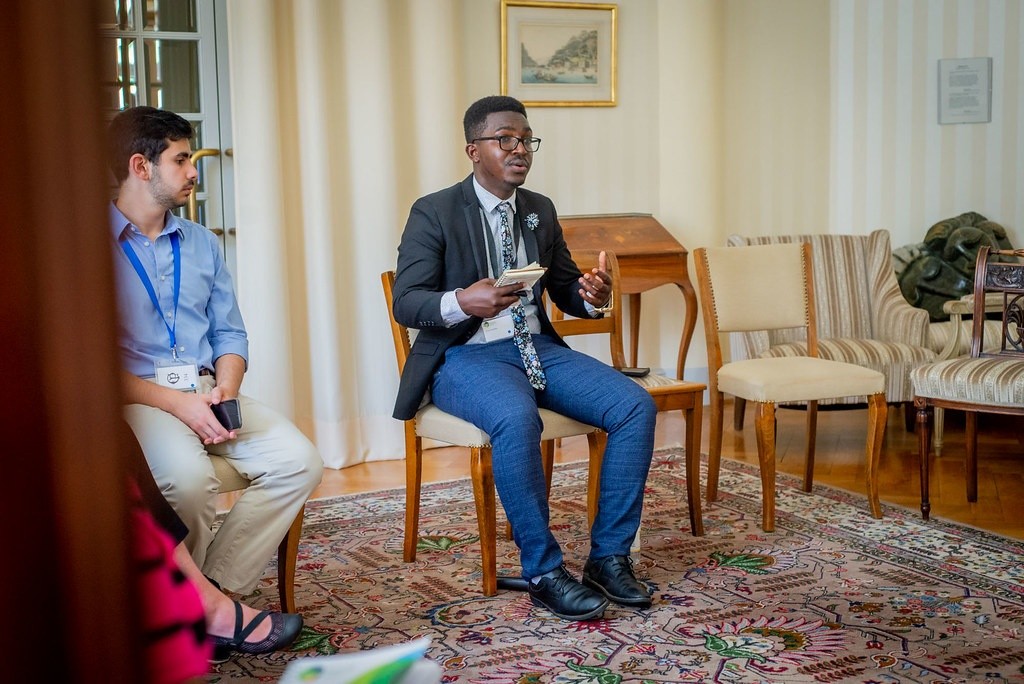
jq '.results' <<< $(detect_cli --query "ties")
[495,205,547,392]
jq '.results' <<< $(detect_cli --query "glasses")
[472,135,541,153]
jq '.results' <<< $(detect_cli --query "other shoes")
[203,601,304,654]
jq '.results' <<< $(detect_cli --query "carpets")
[184,443,1024,684]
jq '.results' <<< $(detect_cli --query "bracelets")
[593,291,613,313]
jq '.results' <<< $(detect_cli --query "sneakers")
[204,645,232,664]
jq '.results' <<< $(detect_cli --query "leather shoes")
[582,553,652,609]
[528,563,610,621]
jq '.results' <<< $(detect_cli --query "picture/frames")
[500,0,620,109]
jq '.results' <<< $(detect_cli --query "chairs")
[204,452,305,614]
[724,228,937,446]
[375,249,707,595]
[692,242,895,533]
[895,242,1024,523]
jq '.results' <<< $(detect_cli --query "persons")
[121,421,303,653]
[392,96,657,621]
[102,105,324,665]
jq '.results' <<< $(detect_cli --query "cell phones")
[211,398,243,431]
[613,368,650,379]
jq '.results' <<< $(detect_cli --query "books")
[493,260,547,294]
